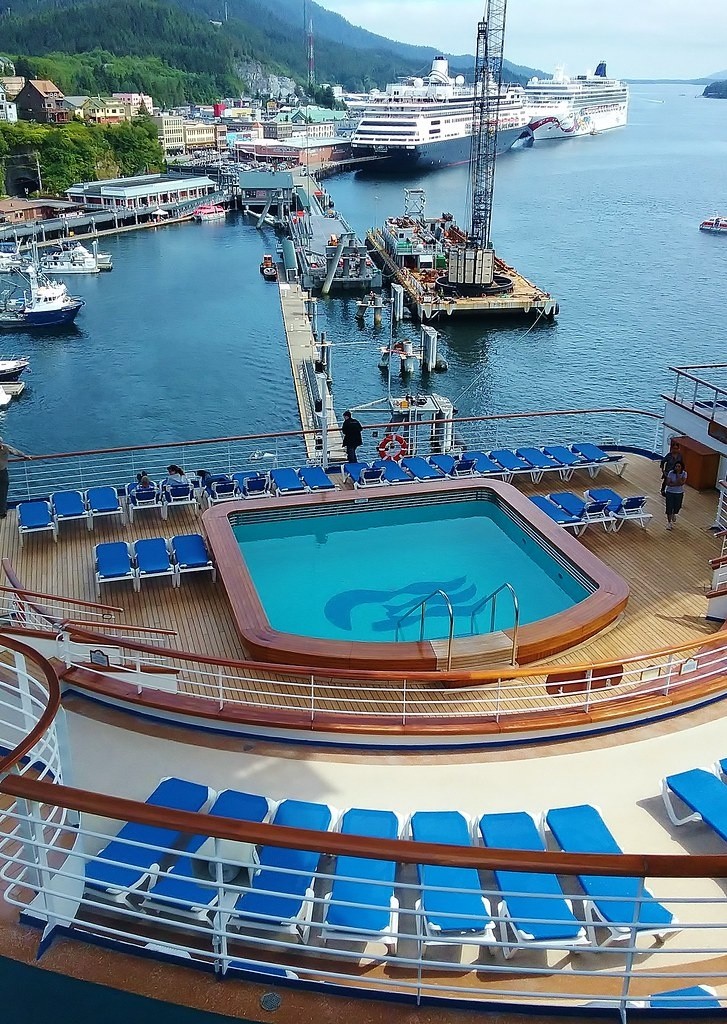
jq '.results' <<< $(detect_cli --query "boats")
[699,215,727,232]
[0,232,119,410]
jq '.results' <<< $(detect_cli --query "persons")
[343,412,364,464]
[665,461,687,530]
[661,442,684,498]
[162,465,191,499]
[129,472,159,501]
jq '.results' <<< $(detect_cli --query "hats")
[141,476,150,484]
[167,465,178,470]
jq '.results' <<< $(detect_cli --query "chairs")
[85,757,727,961]
[645,984,723,1009]
[14,442,653,598]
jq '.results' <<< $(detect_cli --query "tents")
[152,209,168,222]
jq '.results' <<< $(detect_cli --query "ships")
[0,360,727,1024]
[348,80,532,170]
[520,60,629,139]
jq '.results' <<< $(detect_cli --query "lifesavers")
[378,435,407,461]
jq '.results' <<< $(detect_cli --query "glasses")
[169,470,172,473]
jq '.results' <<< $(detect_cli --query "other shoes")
[142,470,147,476]
[672,522,676,530]
[666,521,672,530]
[137,473,141,480]
[660,489,666,497]
